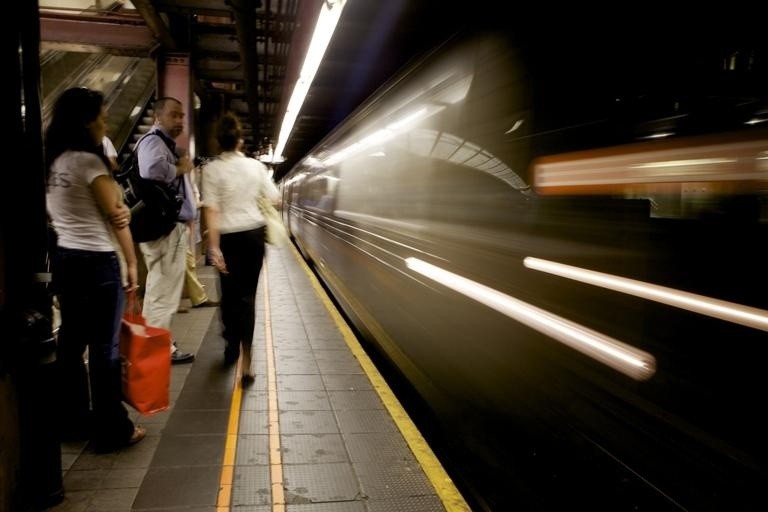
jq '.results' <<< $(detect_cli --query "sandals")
[128,427,146,444]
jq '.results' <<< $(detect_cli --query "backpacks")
[114,129,187,243]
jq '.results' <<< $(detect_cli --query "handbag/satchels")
[256,195,288,247]
[120,316,172,416]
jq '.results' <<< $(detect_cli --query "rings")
[122,219,128,222]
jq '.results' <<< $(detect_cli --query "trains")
[274,0,768,512]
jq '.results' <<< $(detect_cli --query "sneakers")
[192,298,219,308]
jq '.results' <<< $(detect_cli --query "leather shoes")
[169,349,194,365]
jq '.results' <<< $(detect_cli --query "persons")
[39,87,146,456]
[175,146,219,312]
[102,136,119,169]
[250,151,261,160]
[193,156,214,266]
[202,111,282,391]
[132,97,198,364]
